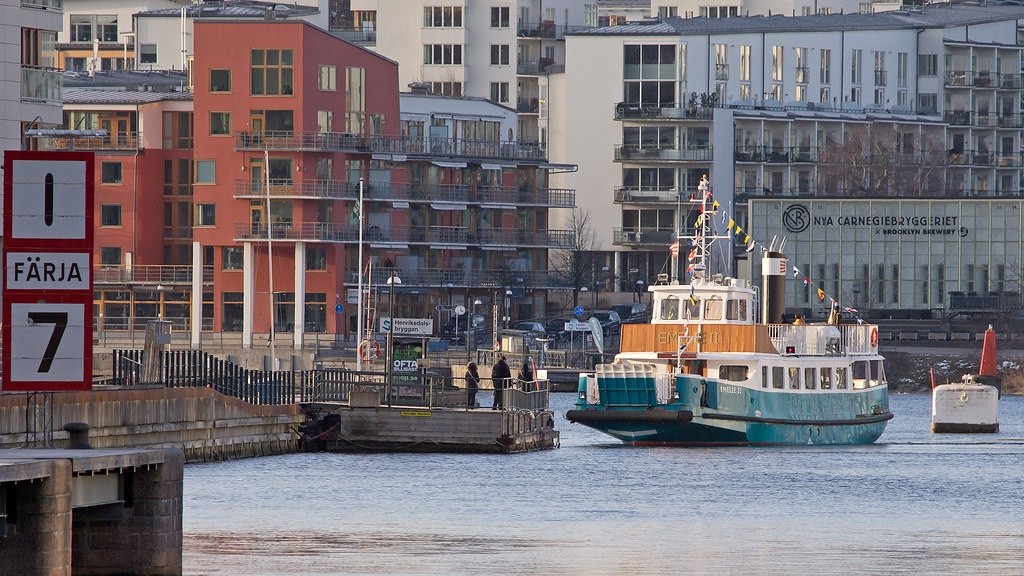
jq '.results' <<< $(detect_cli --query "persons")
[827,306,842,325]
[465,361,480,409]
[492,356,511,410]
[518,364,534,392]
[780,313,792,324]
[792,312,806,325]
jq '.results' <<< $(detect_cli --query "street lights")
[505,289,513,329]
[387,267,402,408]
[467,297,483,363]
[154,282,164,320]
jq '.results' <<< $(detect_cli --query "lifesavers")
[358,340,381,360]
[871,327,878,347]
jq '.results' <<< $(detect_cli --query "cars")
[583,309,622,337]
[545,317,580,347]
[608,303,652,331]
[513,321,547,347]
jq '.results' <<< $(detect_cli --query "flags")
[689,248,697,261]
[669,241,680,256]
[687,309,691,321]
[689,294,698,307]
[687,264,695,276]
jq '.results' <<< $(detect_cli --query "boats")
[565,174,895,447]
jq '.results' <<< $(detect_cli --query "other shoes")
[499,408,506,410]
[492,408,497,410]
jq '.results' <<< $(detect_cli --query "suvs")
[441,312,488,346]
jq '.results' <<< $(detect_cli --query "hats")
[501,356,506,360]
[521,364,530,371]
